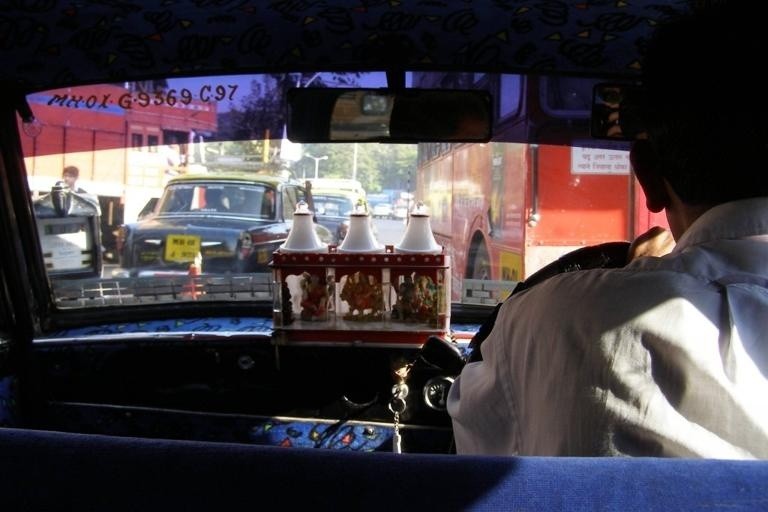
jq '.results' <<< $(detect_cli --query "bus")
[410,70,680,315]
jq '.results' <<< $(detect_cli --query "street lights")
[306,152,330,177]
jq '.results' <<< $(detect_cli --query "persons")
[389,91,491,143]
[62,166,87,195]
[448,0,767,463]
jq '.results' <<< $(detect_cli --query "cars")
[117,176,413,280]
